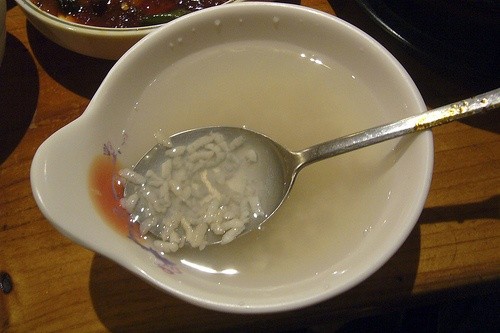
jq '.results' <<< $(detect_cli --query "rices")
[118,127,256,254]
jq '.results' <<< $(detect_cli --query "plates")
[332,1,500,112]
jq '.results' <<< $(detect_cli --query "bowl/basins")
[29,0,435,315]
[16,2,243,61]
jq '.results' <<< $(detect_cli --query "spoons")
[123,89,500,245]
